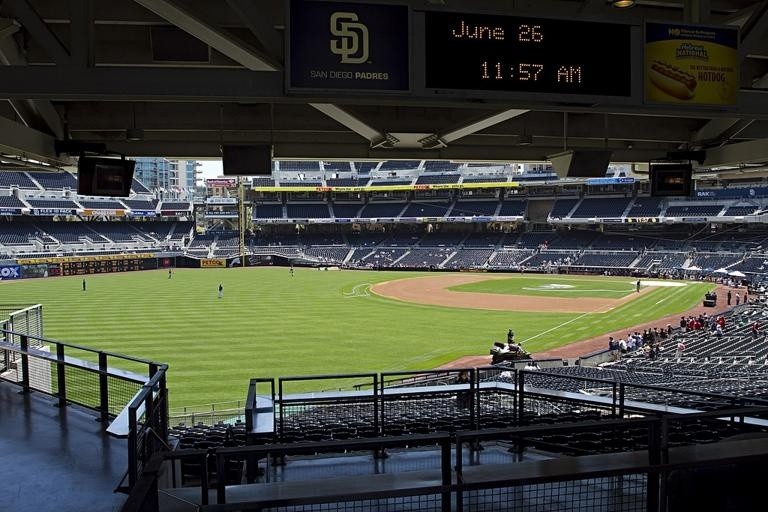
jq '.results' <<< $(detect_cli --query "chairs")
[168,301,768,512]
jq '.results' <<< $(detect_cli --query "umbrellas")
[670,264,744,288]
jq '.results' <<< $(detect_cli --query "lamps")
[126,103,144,141]
[518,113,533,145]
[607,0,637,10]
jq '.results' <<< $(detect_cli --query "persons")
[608,291,768,364]
[523,362,533,370]
[415,244,584,277]
[167,268,171,278]
[636,279,640,292]
[456,371,469,409]
[507,328,514,344]
[289,248,407,277]
[532,363,541,371]
[495,343,525,360]
[217,284,222,298]
